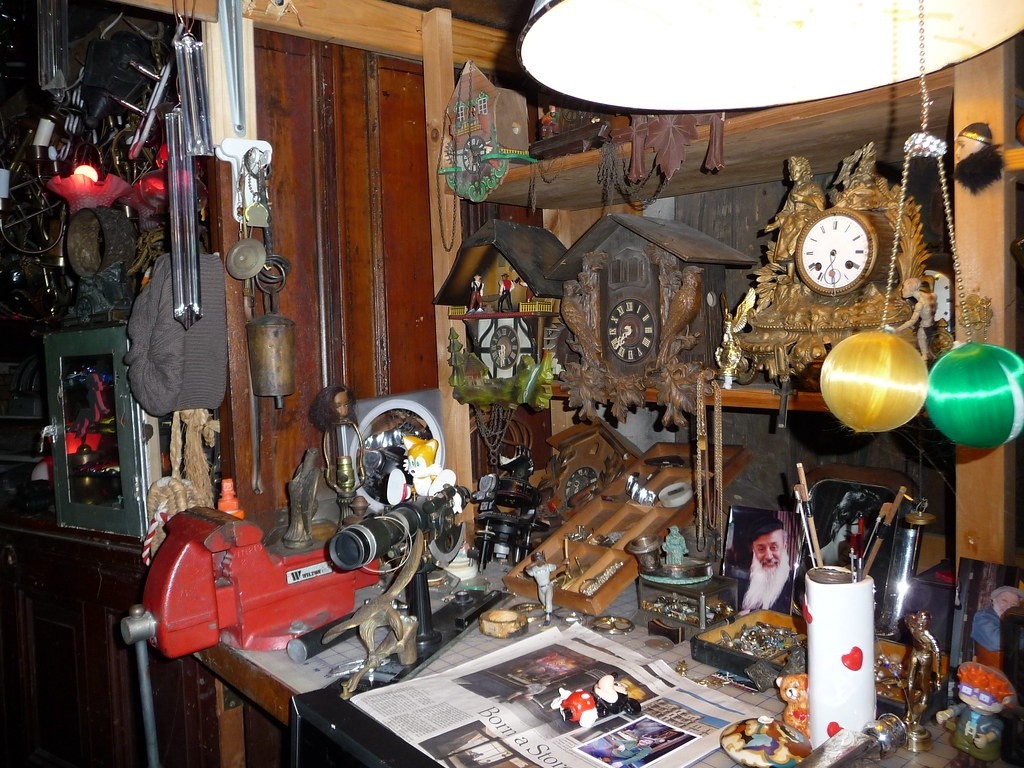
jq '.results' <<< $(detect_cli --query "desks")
[173,560,1012,768]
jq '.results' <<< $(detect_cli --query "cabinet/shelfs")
[0,516,244,768]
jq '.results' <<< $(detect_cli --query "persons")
[662,525,689,565]
[970,585,1021,669]
[905,611,942,729]
[809,488,882,571]
[497,273,515,312]
[468,273,484,313]
[936,662,1017,768]
[526,552,570,627]
[515,277,535,302]
[747,516,803,617]
[588,733,657,768]
[308,384,359,431]
[893,278,937,360]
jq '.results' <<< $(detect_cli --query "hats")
[123,251,229,418]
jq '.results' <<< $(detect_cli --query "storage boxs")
[630,575,739,642]
[693,605,951,731]
[502,440,746,614]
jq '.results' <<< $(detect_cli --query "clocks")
[490,326,518,368]
[710,139,955,393]
[607,299,654,362]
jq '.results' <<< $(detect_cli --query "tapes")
[659,483,693,508]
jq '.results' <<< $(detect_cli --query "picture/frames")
[721,504,805,618]
[795,478,899,639]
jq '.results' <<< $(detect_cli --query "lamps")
[45,143,208,236]
[516,0,1024,112]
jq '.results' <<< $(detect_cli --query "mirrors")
[38,326,163,540]
